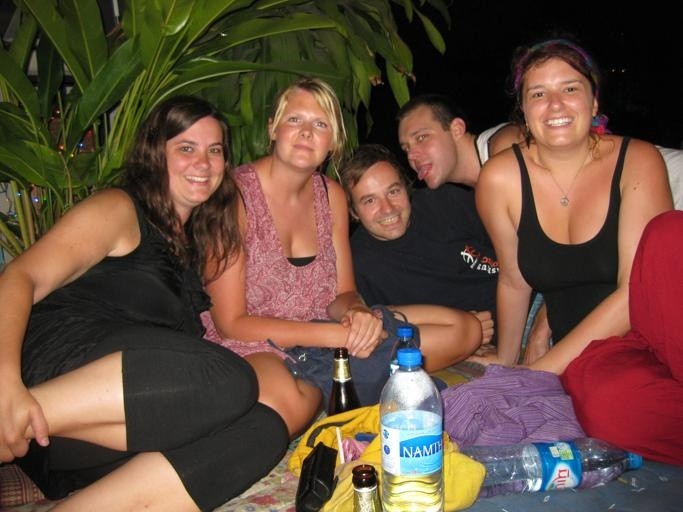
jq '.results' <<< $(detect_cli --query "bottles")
[462,438,642,498]
[353,465,381,511]
[379,327,444,511]
[329,349,362,414]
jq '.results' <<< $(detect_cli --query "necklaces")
[536,150,588,207]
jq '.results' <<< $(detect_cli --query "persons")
[394,91,680,213]
[2,91,292,510]
[338,138,553,356]
[193,76,484,440]
[473,37,683,470]
